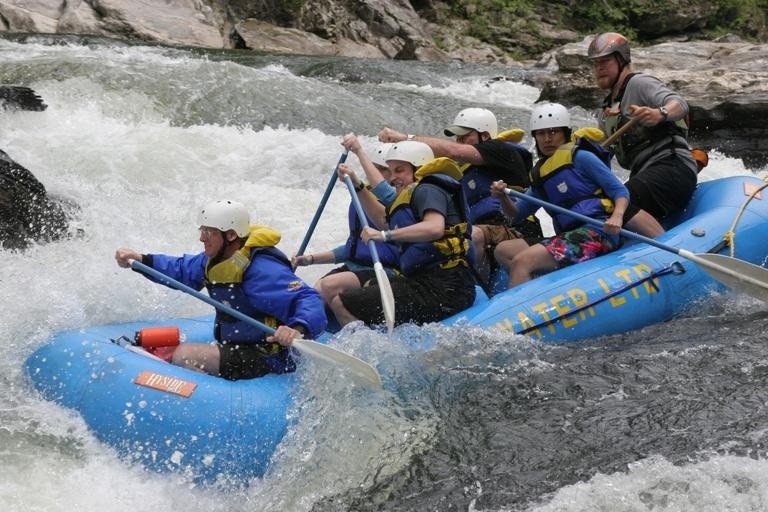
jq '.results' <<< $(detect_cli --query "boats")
[22,174,767,491]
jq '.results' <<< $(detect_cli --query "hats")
[443,126,473,137]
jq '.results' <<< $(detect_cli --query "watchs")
[659,106,669,119]
[381,229,391,243]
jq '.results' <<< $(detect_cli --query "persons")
[378,108,532,292]
[319,140,476,331]
[494,102,632,289]
[585,34,697,239]
[288,133,394,328]
[117,200,325,380]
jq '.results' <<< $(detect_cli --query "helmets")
[386,140,435,166]
[368,142,395,168]
[588,32,630,61]
[453,107,498,140]
[530,103,571,132]
[195,200,250,239]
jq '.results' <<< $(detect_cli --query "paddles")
[342,172,396,340]
[502,186,768,303]
[126,257,383,394]
